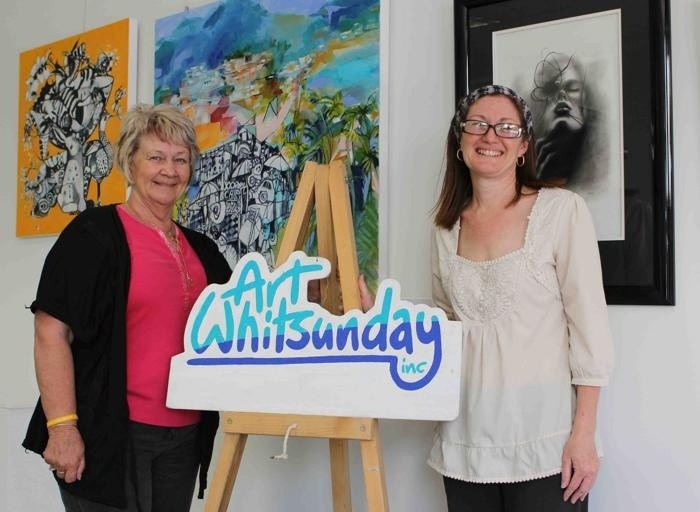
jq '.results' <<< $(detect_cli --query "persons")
[338,86,612,512]
[530,55,589,179]
[22,103,233,512]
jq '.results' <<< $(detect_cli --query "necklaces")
[127,200,191,281]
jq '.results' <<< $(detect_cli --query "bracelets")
[47,414,78,428]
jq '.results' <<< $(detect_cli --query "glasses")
[459,120,525,138]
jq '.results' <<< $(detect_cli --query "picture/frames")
[453,1,672,306]
[13,17,137,237]
[137,0,392,322]
